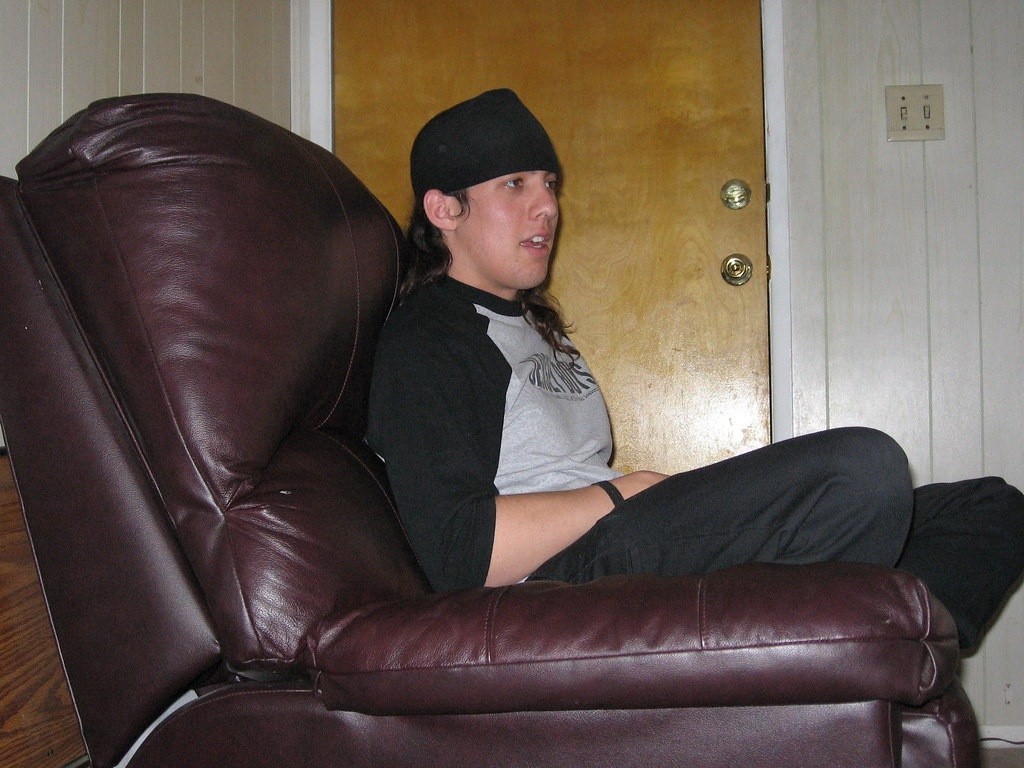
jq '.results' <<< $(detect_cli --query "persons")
[365,85,1024,657]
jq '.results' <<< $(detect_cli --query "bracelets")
[592,481,625,507]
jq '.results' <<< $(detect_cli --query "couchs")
[0,93,982,768]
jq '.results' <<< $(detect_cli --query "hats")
[409,88,559,201]
